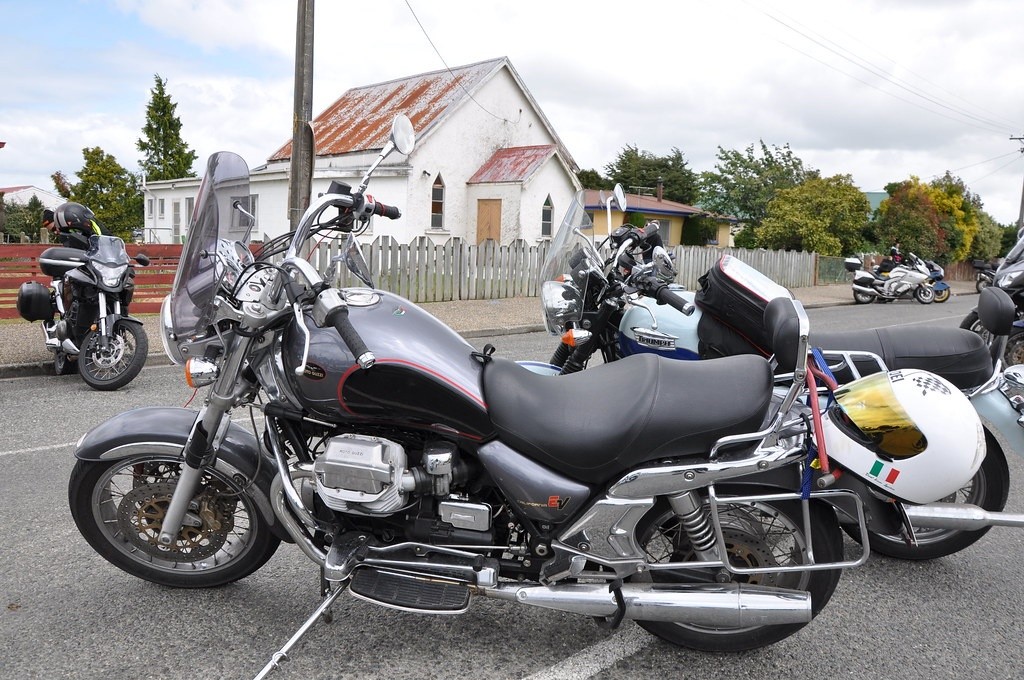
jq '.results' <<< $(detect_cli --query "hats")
[43,210,54,227]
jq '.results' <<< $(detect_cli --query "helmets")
[53,202,94,237]
[812,369,987,506]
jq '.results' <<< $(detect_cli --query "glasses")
[47,224,55,234]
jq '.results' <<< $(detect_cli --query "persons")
[43,209,112,250]
[877,256,896,276]
[890,242,902,260]
[642,219,664,276]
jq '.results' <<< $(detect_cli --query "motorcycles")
[15,209,151,393]
[68,106,871,680]
[537,179,1023,562]
[843,252,935,304]
[871,258,951,303]
[972,259,1000,293]
[958,227,1024,370]
